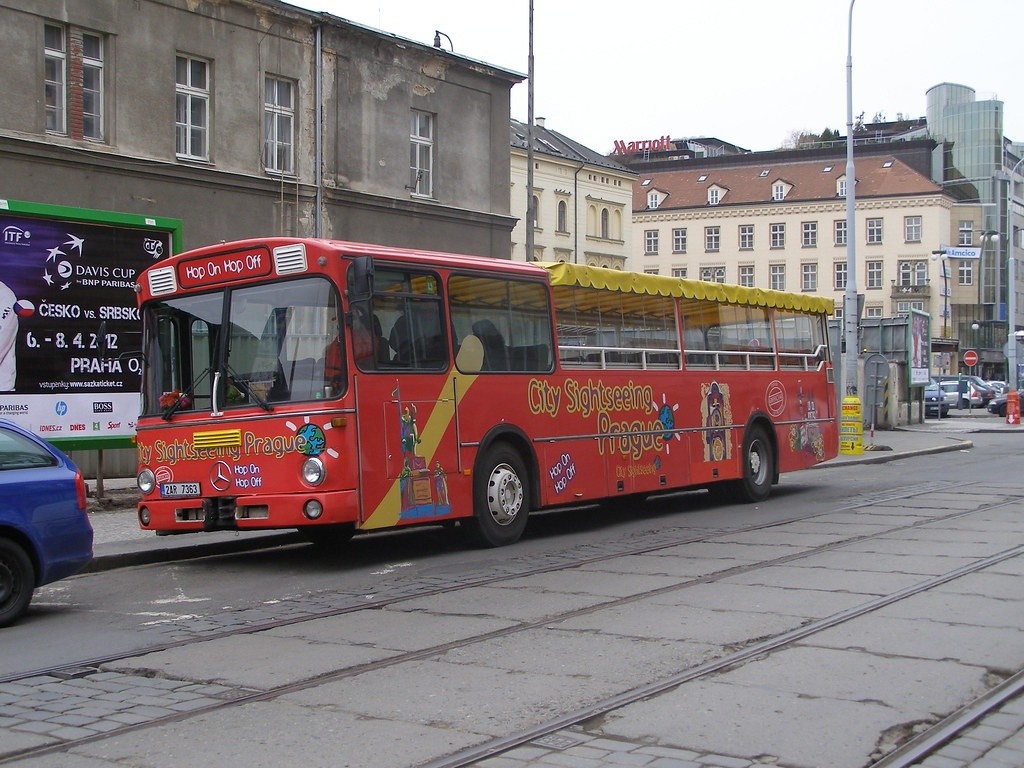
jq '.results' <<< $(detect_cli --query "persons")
[316,302,372,385]
[387,291,458,365]
[469,318,509,371]
[0,278,22,394]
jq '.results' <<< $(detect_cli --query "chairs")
[391,309,817,372]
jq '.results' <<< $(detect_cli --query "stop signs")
[962,349,978,367]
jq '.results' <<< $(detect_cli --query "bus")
[134,232,842,556]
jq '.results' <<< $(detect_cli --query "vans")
[923,376,949,418]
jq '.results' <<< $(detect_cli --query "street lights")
[979,226,1023,389]
[930,249,949,377]
[970,321,984,381]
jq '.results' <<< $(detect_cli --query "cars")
[931,375,1010,406]
[986,388,1024,417]
[0,416,95,624]
[936,381,983,411]
